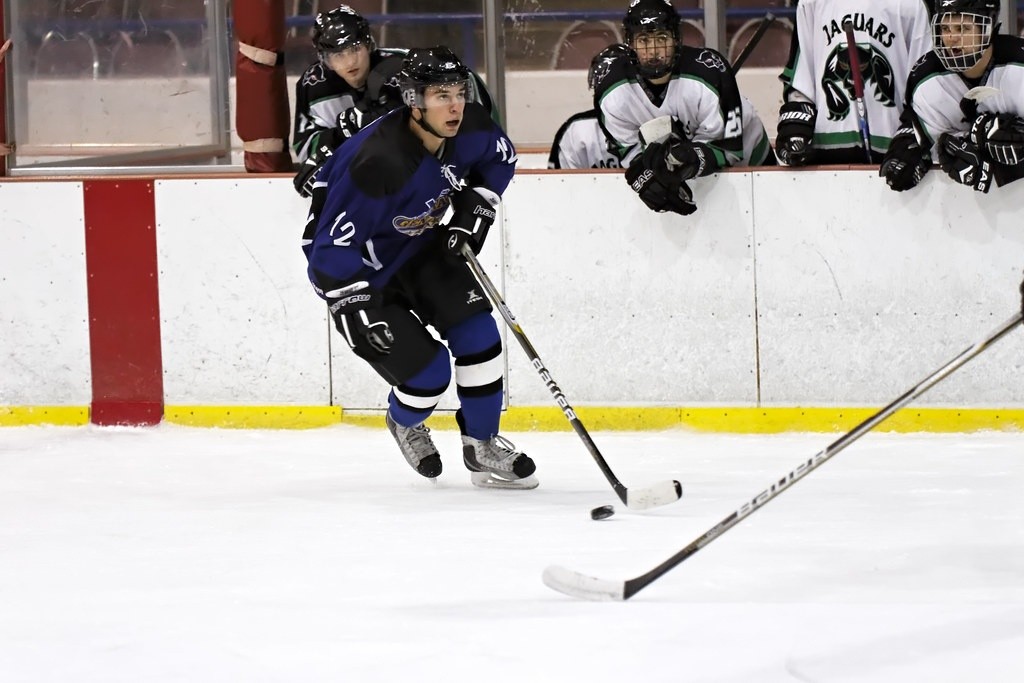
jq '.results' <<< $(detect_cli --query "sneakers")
[384,409,443,485]
[455,407,540,490]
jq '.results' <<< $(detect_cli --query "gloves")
[771,101,818,169]
[336,99,383,139]
[936,110,1024,195]
[327,272,395,365]
[417,185,505,266]
[623,142,699,216]
[657,135,716,187]
[880,122,933,192]
[292,140,342,198]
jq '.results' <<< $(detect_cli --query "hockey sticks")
[960,87,1002,132]
[540,311,1024,603]
[638,114,693,149]
[844,20,875,163]
[459,238,681,508]
[729,11,776,81]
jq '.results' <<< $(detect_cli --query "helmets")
[311,3,376,58]
[622,0,682,79]
[930,0,1003,73]
[398,42,470,93]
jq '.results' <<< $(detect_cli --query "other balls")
[590,505,615,522]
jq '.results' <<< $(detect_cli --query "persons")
[289,4,503,199]
[302,45,540,490]
[905,0,1024,195]
[546,0,782,216]
[775,0,935,192]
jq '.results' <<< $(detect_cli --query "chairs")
[28,17,794,80]
